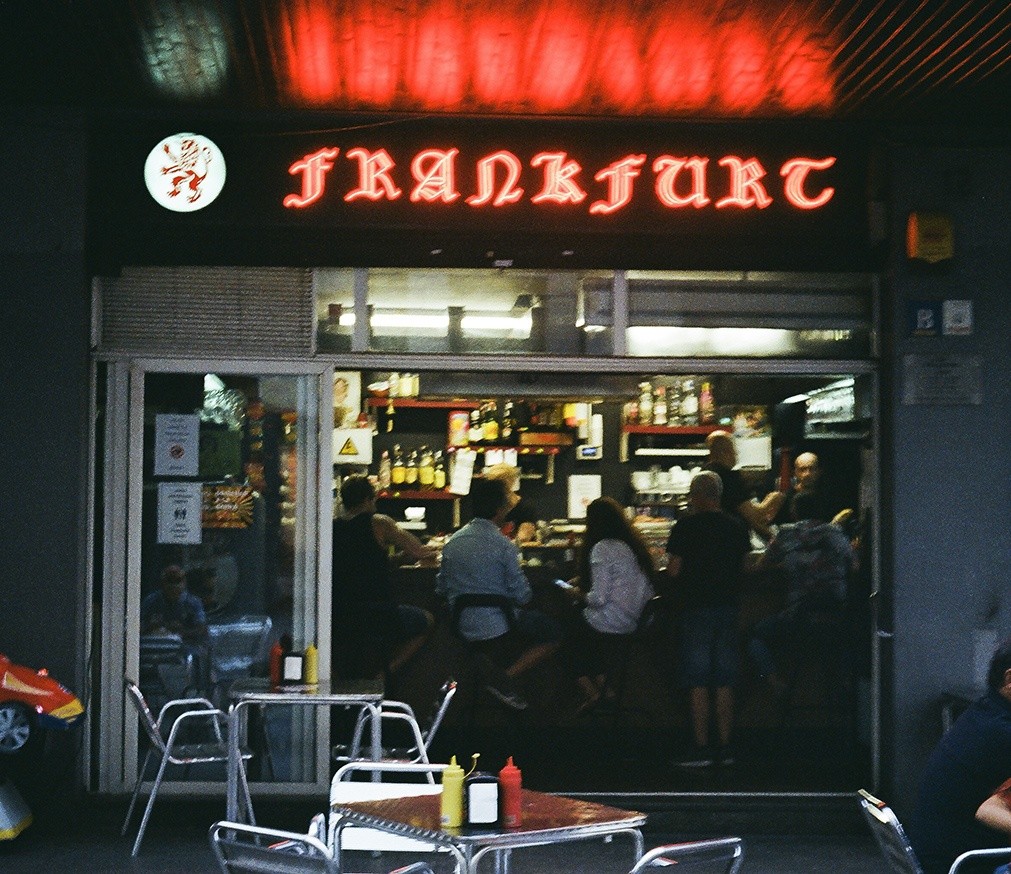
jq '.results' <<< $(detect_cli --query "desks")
[227,678,384,857]
[942,688,984,736]
[331,789,649,874]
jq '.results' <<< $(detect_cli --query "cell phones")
[554,578,574,589]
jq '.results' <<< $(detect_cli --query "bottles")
[638,377,715,428]
[468,397,563,446]
[305,642,317,685]
[270,639,283,682]
[500,756,521,827]
[440,755,464,828]
[378,444,447,492]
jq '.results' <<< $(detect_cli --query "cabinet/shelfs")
[368,399,738,528]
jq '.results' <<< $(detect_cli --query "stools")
[771,601,861,761]
[449,593,667,714]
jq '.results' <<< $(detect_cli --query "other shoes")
[715,751,736,768]
[674,750,712,768]
[486,678,529,711]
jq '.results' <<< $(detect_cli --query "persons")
[913,643,1011,874]
[333,430,865,769]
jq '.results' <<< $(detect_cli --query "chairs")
[121,678,260,856]
[335,680,457,783]
[628,837,746,874]
[858,789,924,874]
[208,820,434,874]
[328,762,467,874]
